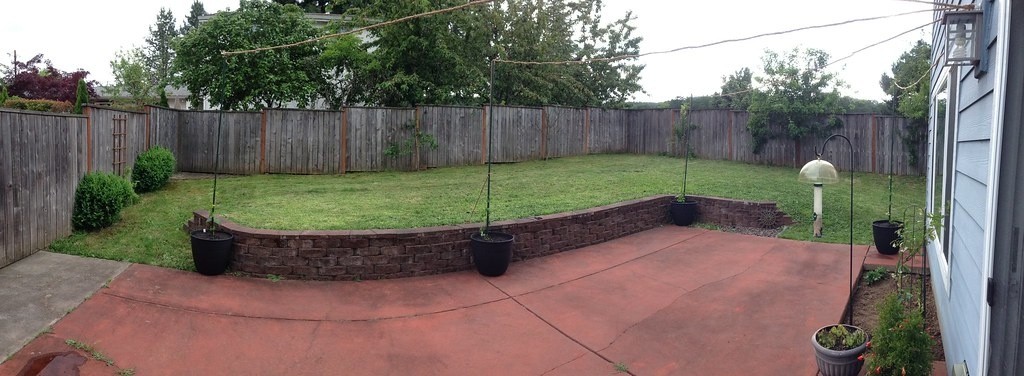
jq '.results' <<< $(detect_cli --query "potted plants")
[812,324,868,376]
[670,103,699,226]
[189,183,233,276]
[871,175,905,255]
[469,201,514,276]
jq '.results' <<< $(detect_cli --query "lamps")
[799,154,839,238]
[942,11,982,66]
